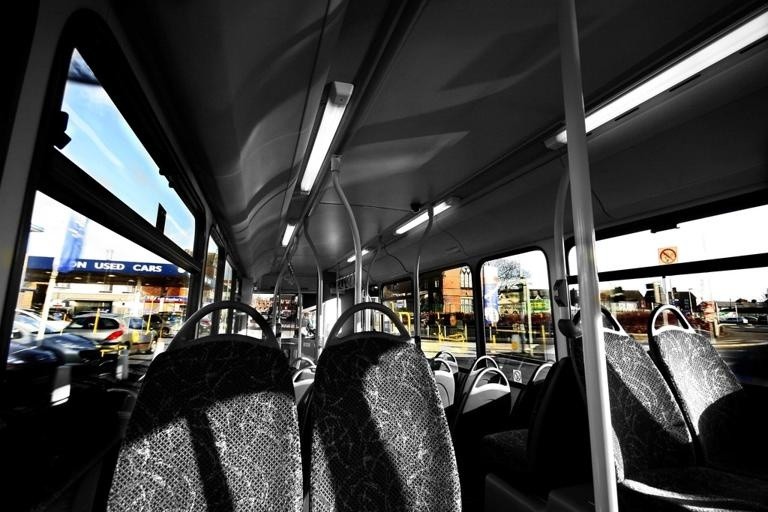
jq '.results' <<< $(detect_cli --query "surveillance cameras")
[410,203,423,212]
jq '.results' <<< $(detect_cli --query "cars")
[717,314,767,326]
[9,303,211,360]
[251,309,289,327]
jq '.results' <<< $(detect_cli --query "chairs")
[290,355,317,405]
[105,299,304,510]
[310,302,463,512]
[476,354,576,506]
[428,351,555,439]
[566,303,767,511]
[646,302,767,485]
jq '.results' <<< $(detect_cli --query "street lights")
[688,286,695,317]
